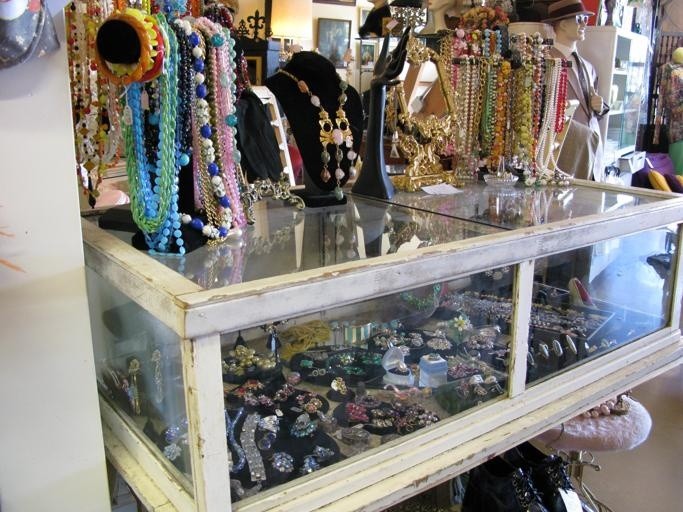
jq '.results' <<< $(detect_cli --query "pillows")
[650,169,683,193]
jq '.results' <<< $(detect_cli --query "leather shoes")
[504,441,583,512]
[459,456,552,511]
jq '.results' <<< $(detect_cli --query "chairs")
[633,152,683,193]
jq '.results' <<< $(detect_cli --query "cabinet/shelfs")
[80,173,683,512]
[549,26,651,283]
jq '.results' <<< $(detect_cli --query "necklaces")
[65,0,568,256]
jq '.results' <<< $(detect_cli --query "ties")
[571,51,589,112]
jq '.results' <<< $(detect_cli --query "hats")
[540,0,595,23]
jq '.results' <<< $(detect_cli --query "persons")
[654,47,683,175]
[541,0,610,292]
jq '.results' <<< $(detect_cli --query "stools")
[533,395,652,511]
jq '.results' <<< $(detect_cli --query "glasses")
[574,15,589,25]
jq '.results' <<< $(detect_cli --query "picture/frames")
[362,45,374,68]
[245,56,263,87]
[317,18,351,67]
[359,7,371,27]
